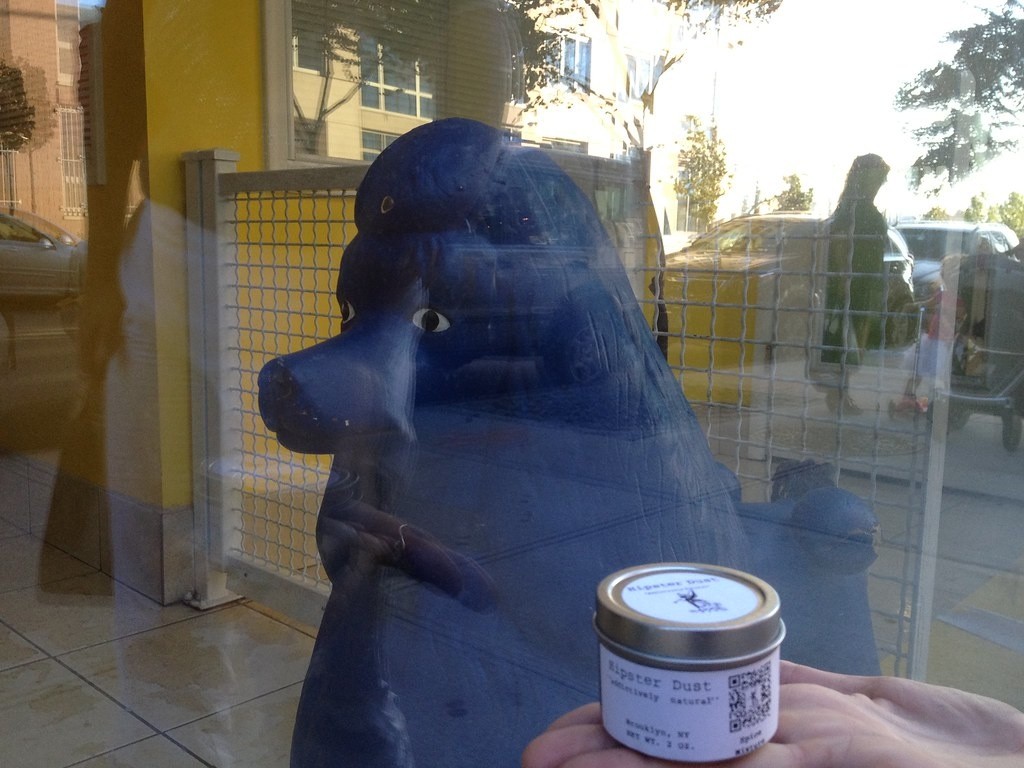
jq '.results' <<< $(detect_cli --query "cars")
[886,218,1020,331]
[0,207,87,309]
[663,213,918,373]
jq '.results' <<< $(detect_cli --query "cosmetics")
[593,563,787,763]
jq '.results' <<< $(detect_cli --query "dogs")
[257,115,883,764]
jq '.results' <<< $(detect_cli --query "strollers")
[889,244,1024,454]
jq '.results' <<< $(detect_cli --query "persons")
[521,657,1024,768]
[898,252,971,415]
[820,153,891,415]
